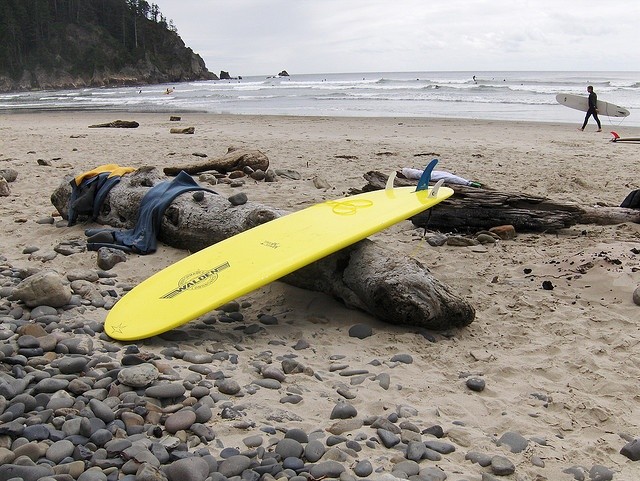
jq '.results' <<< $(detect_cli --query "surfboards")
[104,159,454,342]
[610,131,640,143]
[555,93,630,117]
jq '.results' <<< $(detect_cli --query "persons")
[472,74,476,84]
[578,86,604,133]
